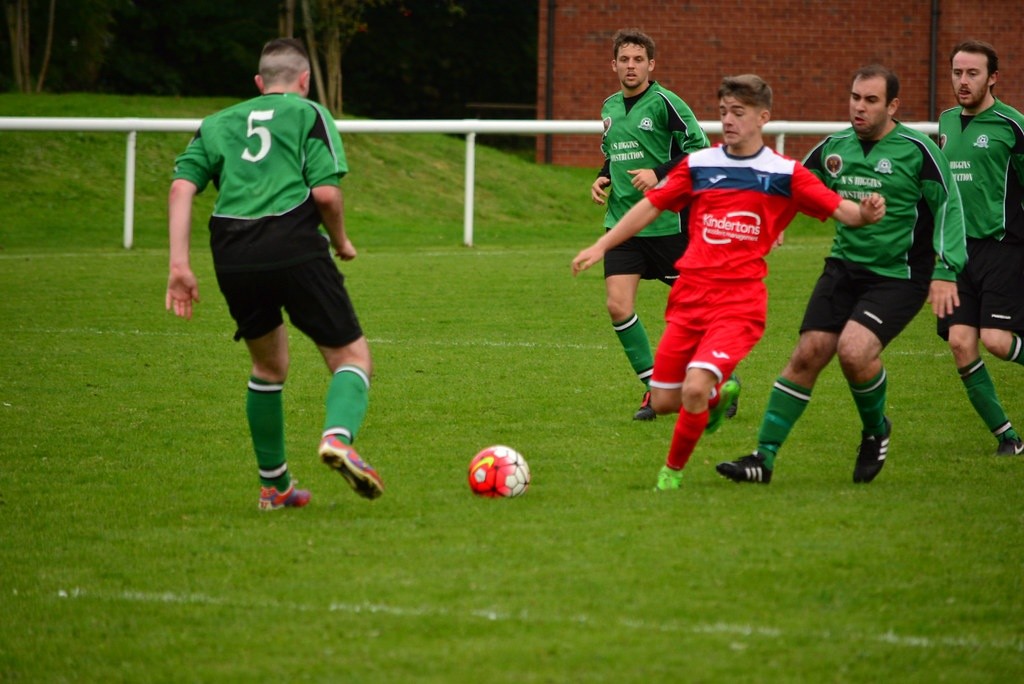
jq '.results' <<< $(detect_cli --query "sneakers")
[725,375,741,418]
[853,415,892,483]
[634,391,656,421]
[705,381,740,436]
[716,451,772,484]
[318,435,384,500]
[653,466,685,494]
[995,436,1024,457]
[257,482,311,512]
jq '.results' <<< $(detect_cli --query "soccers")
[468,444,531,496]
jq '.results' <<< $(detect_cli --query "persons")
[932,43,1024,456]
[571,73,887,489]
[589,27,741,424]
[167,38,383,511]
[714,65,969,484]
